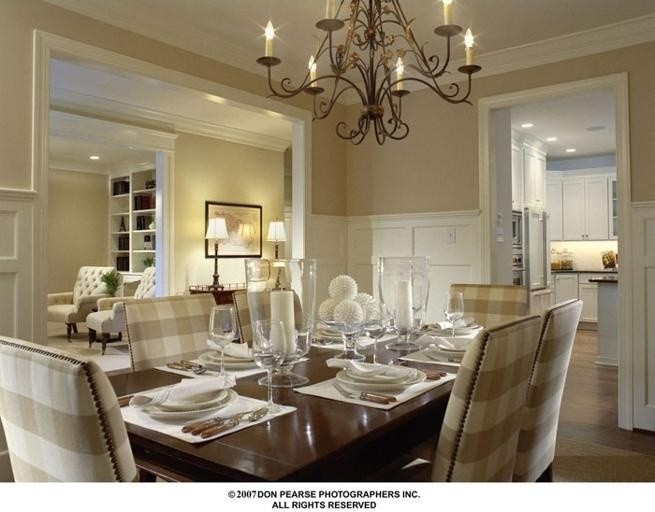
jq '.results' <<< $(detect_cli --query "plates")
[436,324,479,330]
[428,324,484,335]
[434,337,474,351]
[141,388,238,422]
[153,389,228,412]
[430,343,466,358]
[345,368,409,383]
[198,351,258,369]
[207,351,253,362]
[335,364,427,392]
[316,329,344,343]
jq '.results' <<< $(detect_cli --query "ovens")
[513,264,529,287]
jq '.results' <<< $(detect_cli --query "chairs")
[123,292,219,373]
[514,297,586,482]
[399,313,544,482]
[233,289,302,344]
[443,283,531,330]
[1,336,139,482]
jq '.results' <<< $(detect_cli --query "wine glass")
[377,255,432,352]
[364,302,388,364]
[251,318,288,414]
[207,305,238,380]
[244,256,318,389]
[442,290,465,337]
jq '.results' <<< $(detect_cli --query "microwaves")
[511,211,523,248]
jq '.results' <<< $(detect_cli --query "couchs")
[86,266,156,354]
[46,265,115,343]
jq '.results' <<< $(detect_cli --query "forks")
[332,382,389,405]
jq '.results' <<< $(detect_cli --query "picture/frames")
[204,201,262,258]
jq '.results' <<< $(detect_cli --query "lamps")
[254,0,480,147]
[205,216,228,290]
[265,221,286,259]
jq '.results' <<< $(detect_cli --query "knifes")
[423,352,462,363]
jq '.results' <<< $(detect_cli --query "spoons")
[166,363,208,375]
[200,405,270,439]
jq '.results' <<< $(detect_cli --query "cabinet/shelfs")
[510,145,522,209]
[579,274,600,323]
[107,164,155,273]
[524,148,546,207]
[512,209,553,313]
[562,178,608,241]
[189,283,246,304]
[547,180,562,241]
[594,282,619,367]
[610,178,619,239]
[557,274,578,303]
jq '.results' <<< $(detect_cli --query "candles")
[460,26,476,65]
[269,284,295,356]
[262,20,275,56]
[394,55,408,89]
[307,54,317,88]
[326,0,335,19]
[396,275,412,330]
[441,0,454,24]
